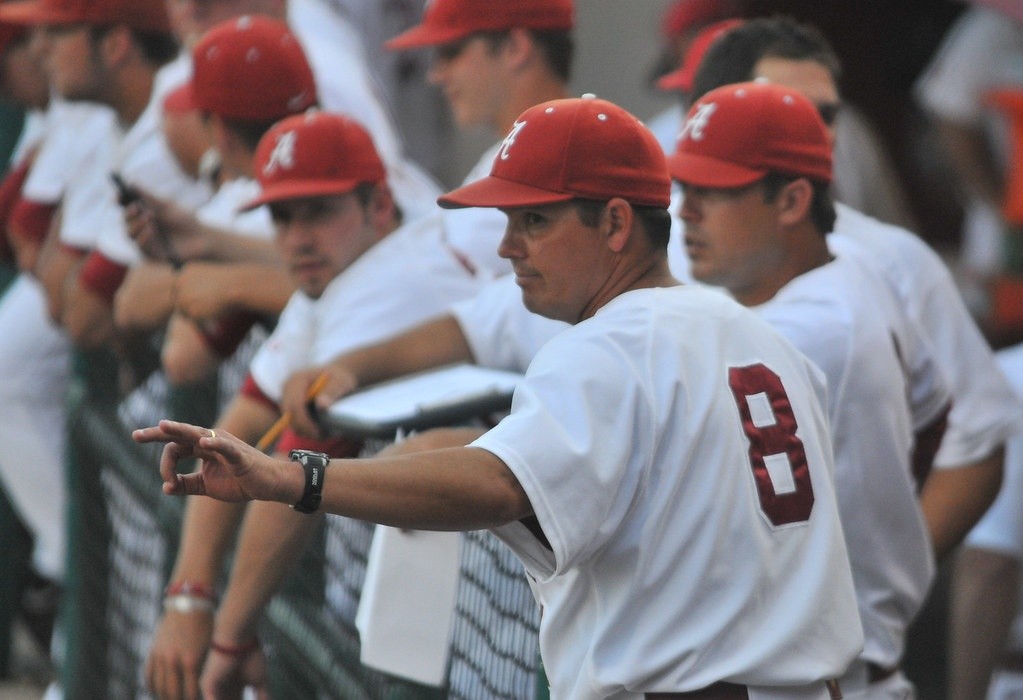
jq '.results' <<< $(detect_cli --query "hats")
[659,17,751,90]
[670,76,834,187]
[163,16,317,122]
[238,108,385,212]
[437,94,673,208]
[3,0,169,33]
[390,0,573,51]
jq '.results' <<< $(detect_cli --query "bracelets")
[160,595,214,613]
[163,581,217,600]
[207,640,242,658]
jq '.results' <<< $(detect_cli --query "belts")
[647,680,841,700]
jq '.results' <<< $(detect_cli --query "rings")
[208,429,216,438]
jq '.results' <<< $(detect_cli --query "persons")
[0,1,598,700]
[132,94,865,700]
[352,0,1023,700]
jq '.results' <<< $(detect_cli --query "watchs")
[288,449,329,513]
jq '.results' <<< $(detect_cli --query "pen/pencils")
[447,240,477,275]
[254,371,336,451]
